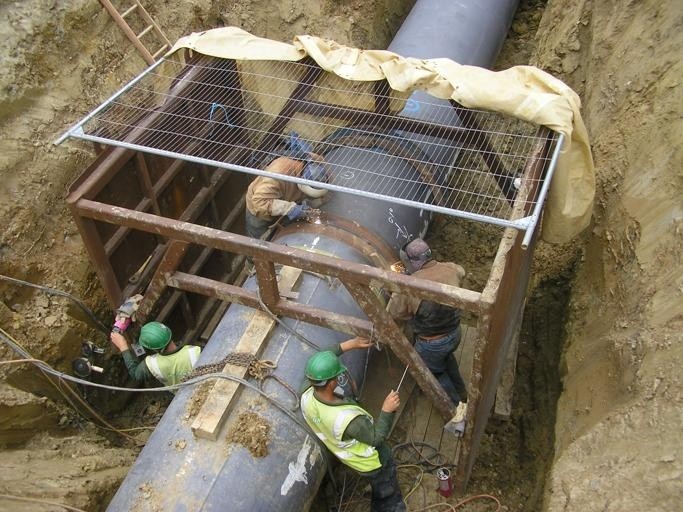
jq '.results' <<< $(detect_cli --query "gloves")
[288,205,305,220]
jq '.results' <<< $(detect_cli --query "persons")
[389,237,468,407]
[296,336,407,512]
[110,320,203,397]
[243,151,335,278]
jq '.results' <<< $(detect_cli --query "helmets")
[138,321,172,350]
[304,351,347,381]
